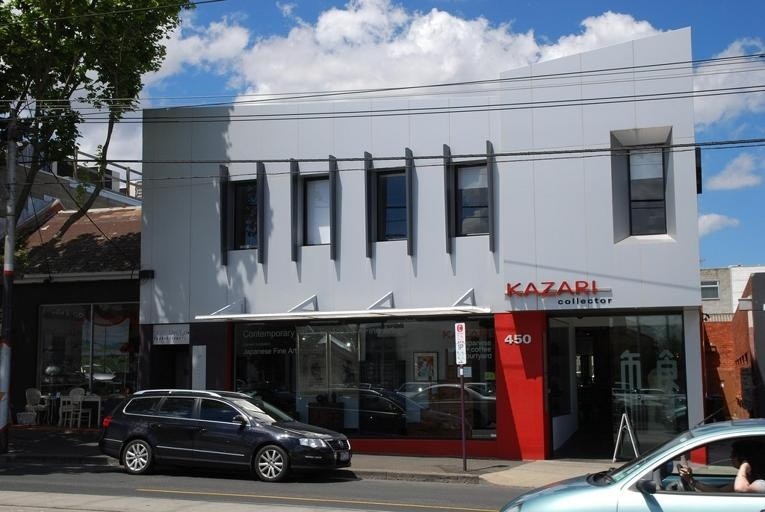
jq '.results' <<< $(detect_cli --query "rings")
[688,472,692,476]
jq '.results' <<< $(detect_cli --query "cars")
[99,388,353,483]
[307,381,496,440]
[80,363,117,383]
[498,418,764,511]
[611,382,687,429]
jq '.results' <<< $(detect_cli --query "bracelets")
[689,478,697,489]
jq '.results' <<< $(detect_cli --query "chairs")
[25,387,102,430]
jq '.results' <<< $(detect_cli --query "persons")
[677,440,765,494]
